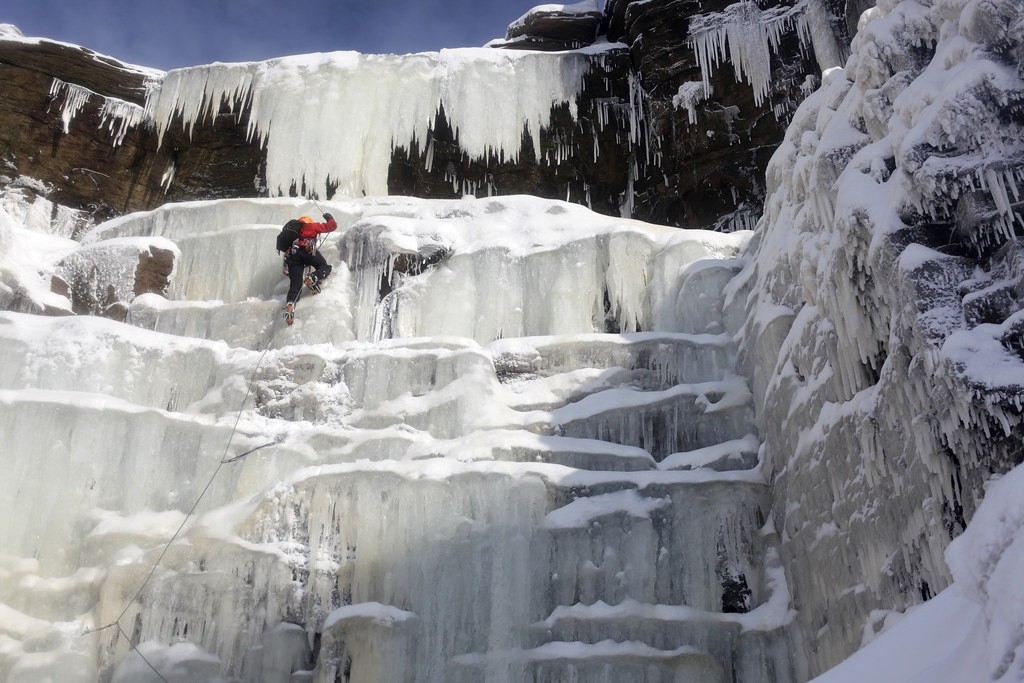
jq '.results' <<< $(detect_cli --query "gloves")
[323,213,333,222]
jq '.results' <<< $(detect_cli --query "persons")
[274,212,338,328]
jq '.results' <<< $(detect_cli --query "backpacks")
[276,219,316,256]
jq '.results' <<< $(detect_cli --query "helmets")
[298,216,314,223]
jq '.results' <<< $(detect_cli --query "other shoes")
[285,305,293,325]
[304,274,321,295]
